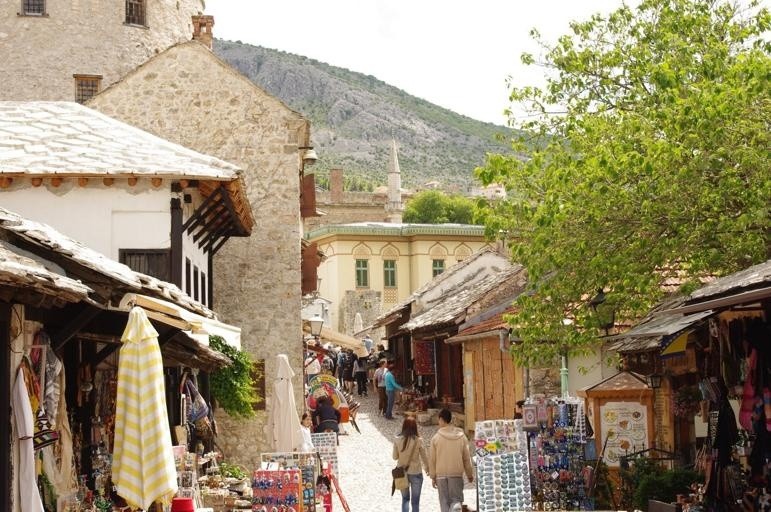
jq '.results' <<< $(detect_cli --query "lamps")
[303,313,325,343]
[643,374,662,388]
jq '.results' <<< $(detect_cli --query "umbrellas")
[110,306,178,512]
[267,354,305,452]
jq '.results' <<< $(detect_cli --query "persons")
[301,412,316,452]
[308,400,341,446]
[306,334,375,399]
[428,407,474,512]
[392,415,430,512]
[384,363,409,419]
[373,357,388,416]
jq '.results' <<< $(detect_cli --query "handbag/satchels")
[191,394,214,436]
[392,466,410,490]
